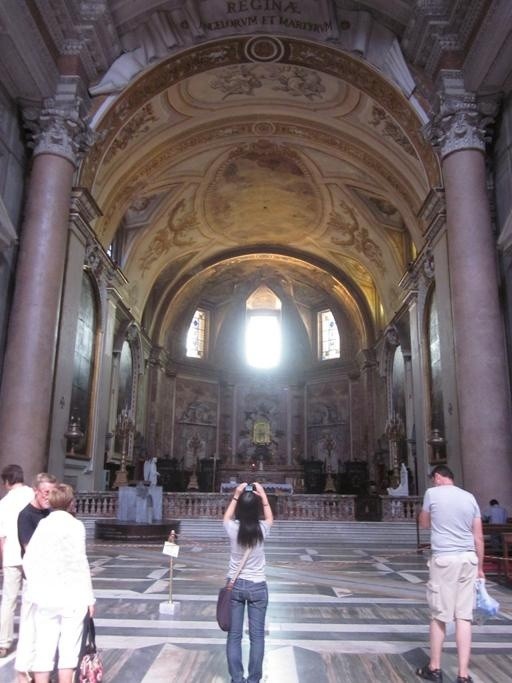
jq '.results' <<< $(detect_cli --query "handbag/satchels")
[73,615,105,682]
[217,585,233,630]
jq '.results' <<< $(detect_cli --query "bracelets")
[264,503,269,506]
[233,498,238,501]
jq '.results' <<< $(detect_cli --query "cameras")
[244,484,255,492]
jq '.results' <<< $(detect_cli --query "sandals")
[416,662,444,682]
[457,674,474,682]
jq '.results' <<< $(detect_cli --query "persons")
[0,464,35,657]
[150,457,159,486]
[483,499,507,556]
[415,466,485,683]
[14,473,57,683]
[22,484,97,683]
[223,482,274,683]
